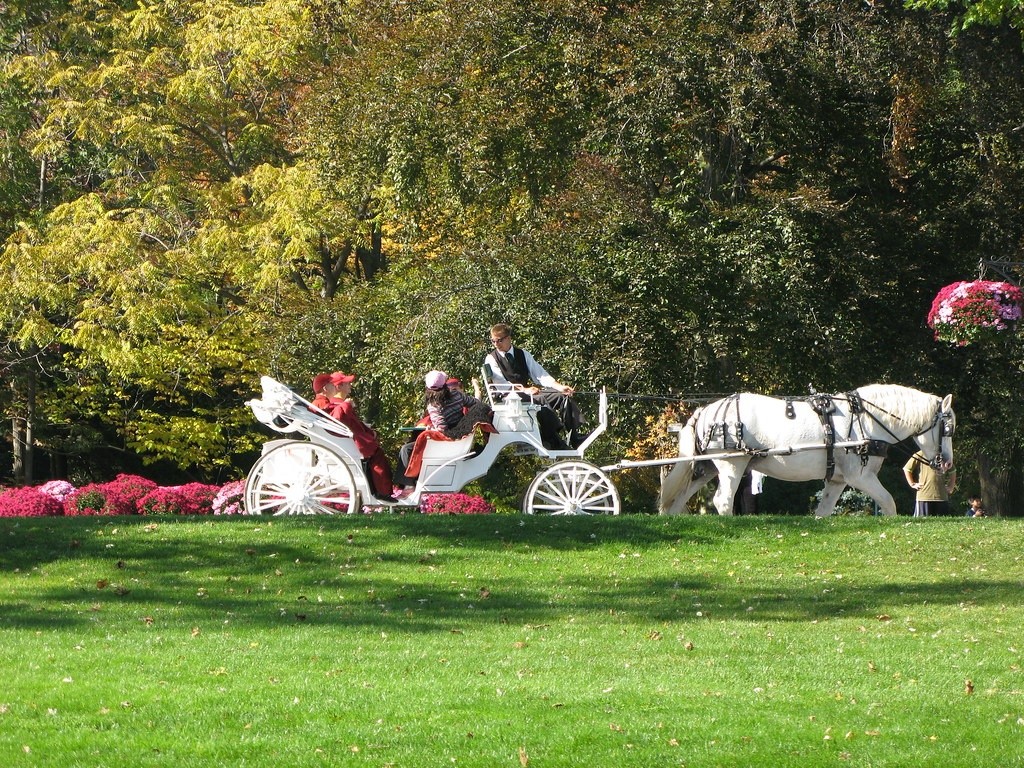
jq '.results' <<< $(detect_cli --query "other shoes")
[377,494,398,503]
[544,437,572,450]
[570,432,588,448]
[394,487,415,500]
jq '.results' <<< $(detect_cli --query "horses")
[660,382,957,516]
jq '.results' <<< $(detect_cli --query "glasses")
[491,334,509,343]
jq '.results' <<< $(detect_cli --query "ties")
[505,353,515,364]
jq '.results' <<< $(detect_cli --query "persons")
[903,449,957,516]
[485,324,592,450]
[308,370,483,503]
[965,495,987,516]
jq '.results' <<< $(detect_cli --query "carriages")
[242,365,955,518]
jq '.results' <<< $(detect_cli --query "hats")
[446,378,458,384]
[425,370,448,390]
[313,375,344,392]
[330,373,354,386]
